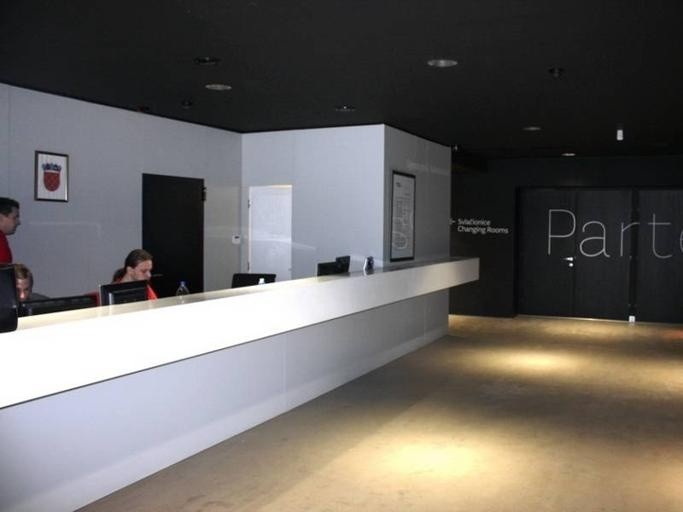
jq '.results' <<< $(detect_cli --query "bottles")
[176,281,189,295]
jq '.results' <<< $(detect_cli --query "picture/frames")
[34,150,69,202]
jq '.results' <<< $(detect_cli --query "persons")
[11,262,50,301]
[96,250,159,305]
[0,197,21,264]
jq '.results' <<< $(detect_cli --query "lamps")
[195,55,233,91]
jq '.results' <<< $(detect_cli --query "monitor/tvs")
[100,279,149,306]
[231,273,277,288]
[19,294,97,317]
[336,255,350,265]
[317,262,349,275]
